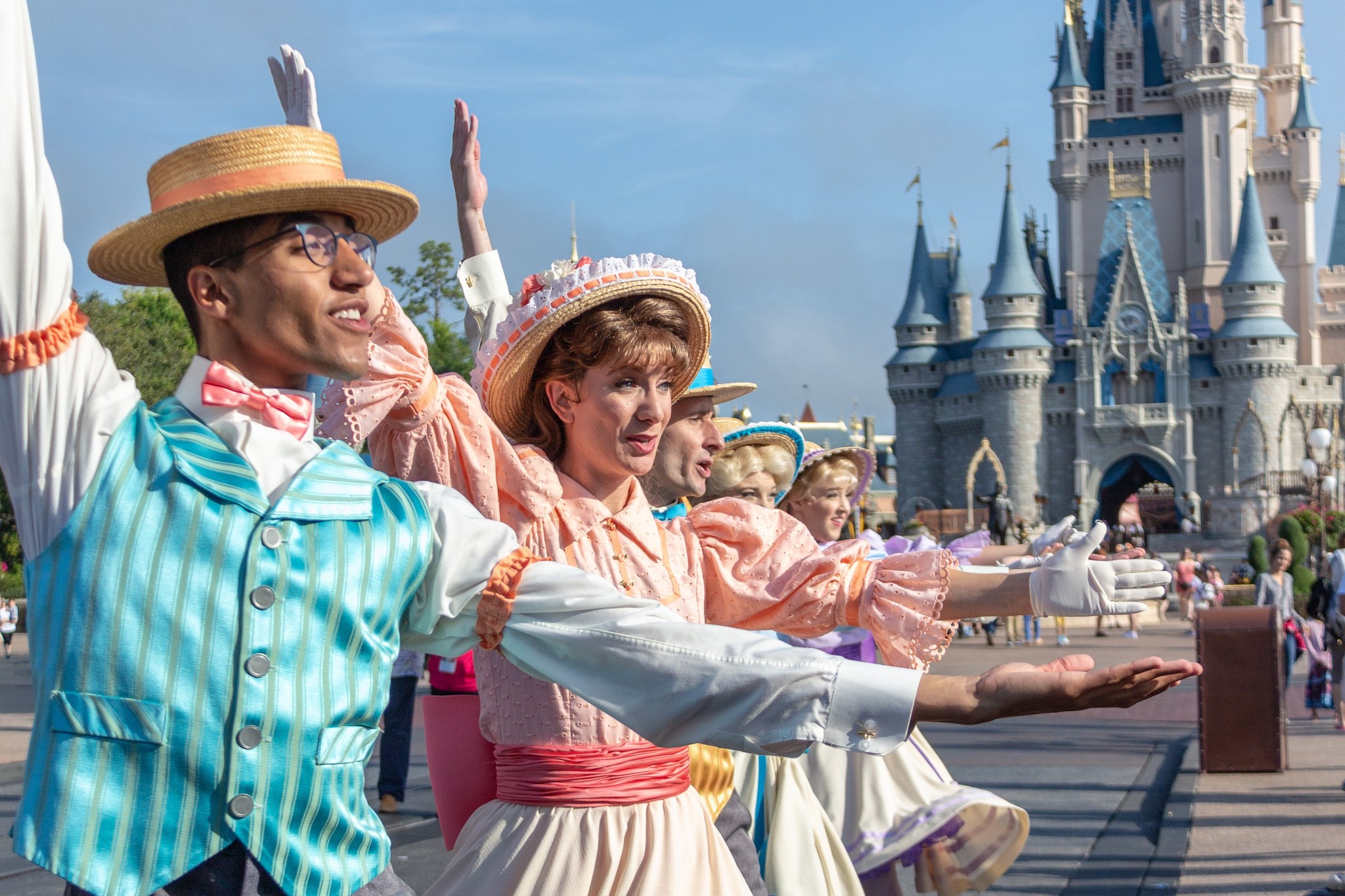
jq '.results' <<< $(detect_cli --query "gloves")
[1003,552,1054,568]
[1027,513,1082,556]
[267,42,323,131]
[1028,521,1172,617]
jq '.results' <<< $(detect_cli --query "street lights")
[1298,428,1337,581]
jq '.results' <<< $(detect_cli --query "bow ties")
[201,360,313,440]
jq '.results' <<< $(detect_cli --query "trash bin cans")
[1197,605,1288,776]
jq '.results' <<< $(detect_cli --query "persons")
[1252,528,1345,731]
[974,478,1016,545]
[377,99,1146,896]
[0,598,18,658]
[0,0,1204,896]
[269,47,1172,896]
[1173,488,1255,637]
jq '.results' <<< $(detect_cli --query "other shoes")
[378,794,399,812]
[1057,634,1070,646]
[1026,637,1044,646]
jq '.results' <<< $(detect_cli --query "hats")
[469,252,712,440]
[798,441,877,511]
[711,415,805,510]
[680,345,757,408]
[87,125,419,288]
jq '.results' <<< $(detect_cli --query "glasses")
[205,222,379,270]
